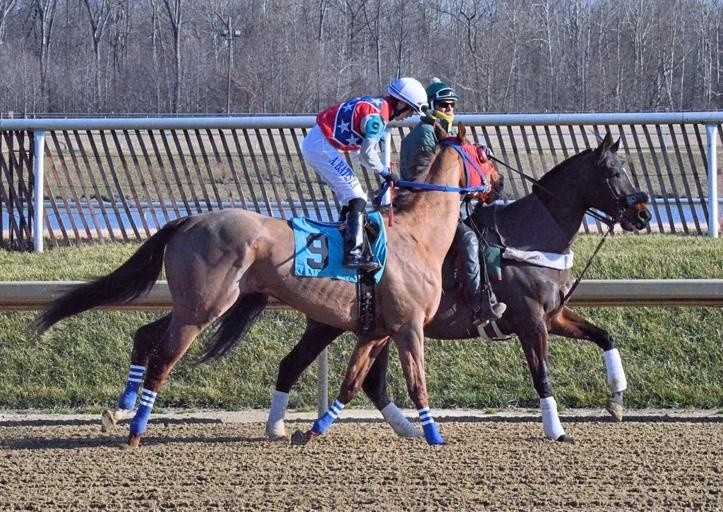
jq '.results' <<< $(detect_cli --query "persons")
[302,77,428,271]
[400,76,506,323]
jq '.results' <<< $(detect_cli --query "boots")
[457,231,507,325]
[343,211,379,269]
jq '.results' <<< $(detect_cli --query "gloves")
[379,167,399,186]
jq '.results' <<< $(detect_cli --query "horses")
[28,119,504,449]
[189,132,652,446]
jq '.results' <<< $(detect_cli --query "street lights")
[219,17,241,113]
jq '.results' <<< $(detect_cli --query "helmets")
[388,77,429,117]
[426,77,460,110]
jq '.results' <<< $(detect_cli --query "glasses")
[435,102,455,108]
